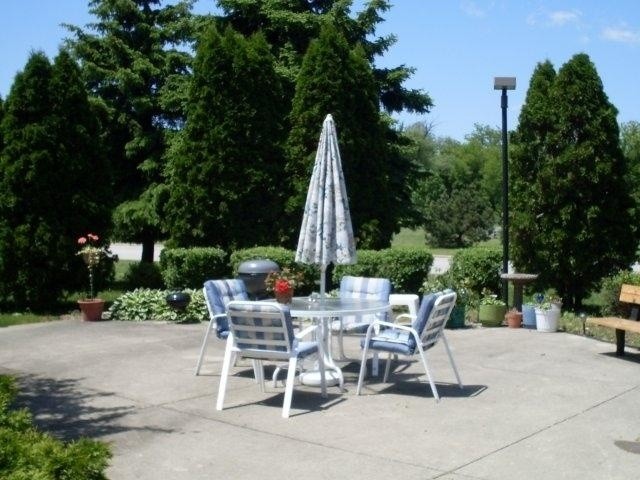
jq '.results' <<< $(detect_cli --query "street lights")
[494,76,517,309]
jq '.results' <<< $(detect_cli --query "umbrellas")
[292,110,361,371]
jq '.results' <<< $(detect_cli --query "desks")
[500,273,539,312]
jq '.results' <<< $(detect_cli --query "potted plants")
[478,288,563,333]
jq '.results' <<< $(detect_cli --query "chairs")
[193,275,464,420]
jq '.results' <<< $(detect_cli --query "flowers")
[76,233,106,299]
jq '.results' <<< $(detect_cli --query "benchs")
[590,283,640,355]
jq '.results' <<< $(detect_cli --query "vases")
[78,297,106,323]
